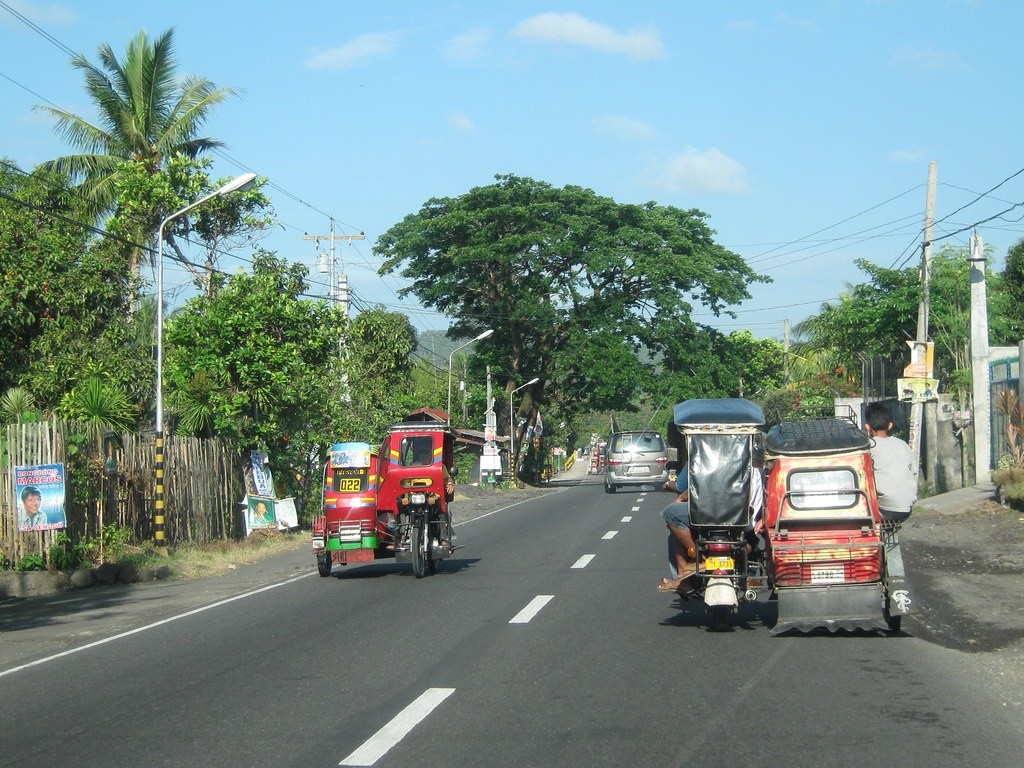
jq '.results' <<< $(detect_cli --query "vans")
[606,429,670,495]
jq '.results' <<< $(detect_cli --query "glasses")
[417,450,430,454]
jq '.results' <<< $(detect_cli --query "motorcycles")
[312,419,463,581]
[655,396,893,636]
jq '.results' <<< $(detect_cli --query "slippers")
[673,566,705,583]
[657,582,696,594]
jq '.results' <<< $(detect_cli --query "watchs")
[447,481,454,485]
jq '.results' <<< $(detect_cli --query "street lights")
[510,375,540,488]
[446,329,497,431]
[154,170,259,555]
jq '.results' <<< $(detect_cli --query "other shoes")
[442,540,451,554]
[689,548,697,558]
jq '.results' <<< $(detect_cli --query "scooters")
[871,515,917,632]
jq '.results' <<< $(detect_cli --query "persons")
[654,454,764,590]
[252,501,273,526]
[403,439,455,495]
[864,403,918,524]
[903,342,932,377]
[922,382,937,397]
[18,486,47,529]
[902,382,914,398]
